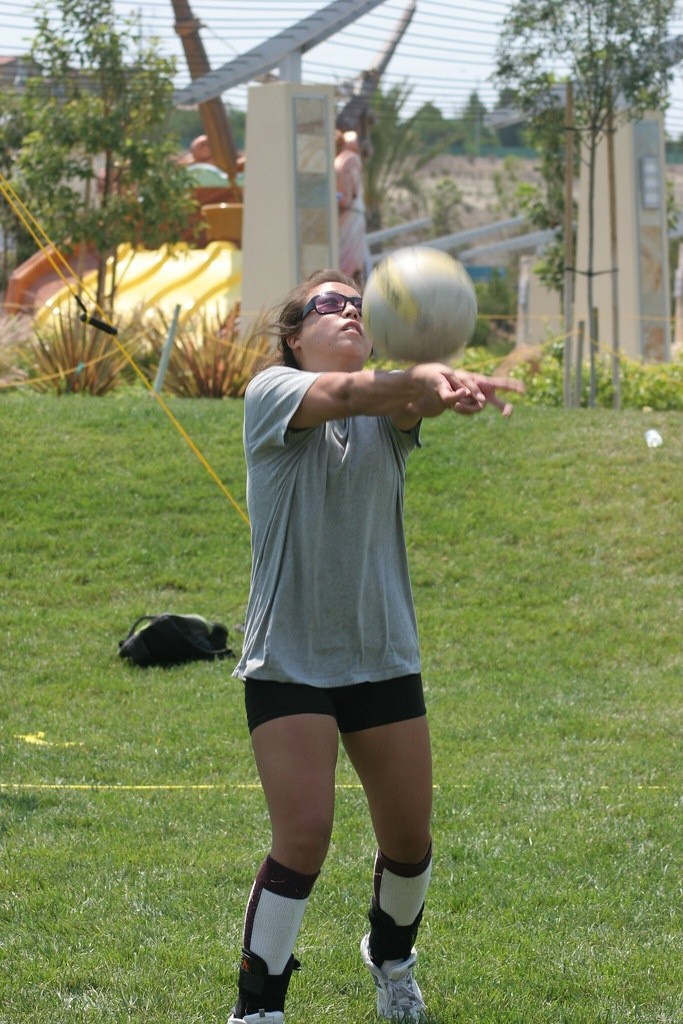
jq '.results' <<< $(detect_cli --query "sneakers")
[227,1009,285,1024]
[360,929,428,1024]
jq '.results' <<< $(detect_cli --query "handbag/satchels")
[118,614,236,669]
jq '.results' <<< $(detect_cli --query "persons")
[231,268,526,1023]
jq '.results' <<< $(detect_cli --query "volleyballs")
[362,246,483,364]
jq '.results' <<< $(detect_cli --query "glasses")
[298,292,364,321]
